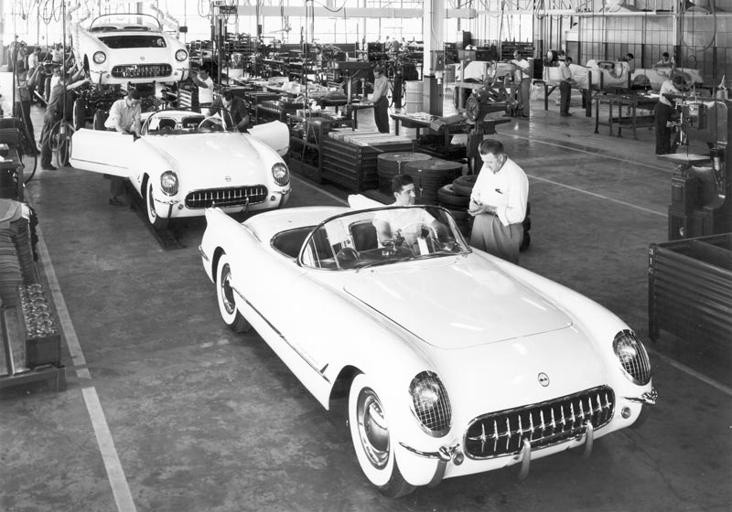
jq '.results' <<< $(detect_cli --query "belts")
[482,211,500,219]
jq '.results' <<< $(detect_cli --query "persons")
[1,31,689,207]
[375,174,449,249]
[466,139,530,265]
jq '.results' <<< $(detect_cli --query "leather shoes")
[109,197,123,206]
[42,165,56,171]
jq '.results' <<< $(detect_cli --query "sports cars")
[67,9,192,98]
[66,107,292,232]
[194,192,661,502]
